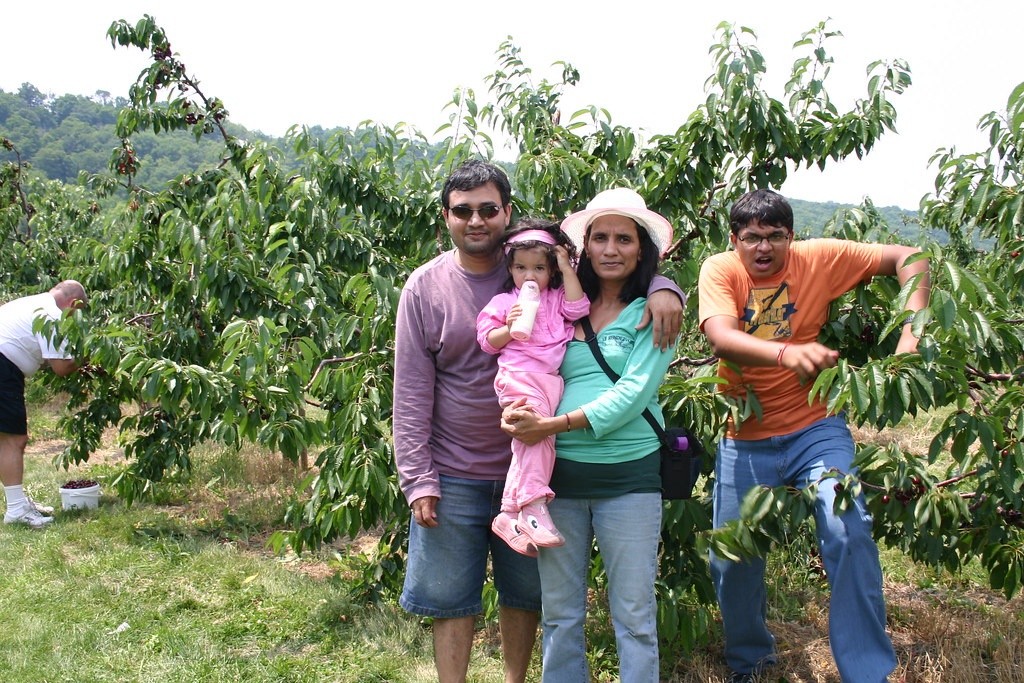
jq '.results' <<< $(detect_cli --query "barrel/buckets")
[59,483,103,511]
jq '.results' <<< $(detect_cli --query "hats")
[560,187,674,264]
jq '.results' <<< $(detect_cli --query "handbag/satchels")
[656,425,715,500]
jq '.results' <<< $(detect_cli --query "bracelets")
[565,414,570,432]
[902,313,916,324]
[777,344,792,366]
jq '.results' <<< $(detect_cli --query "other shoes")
[3,497,54,528]
[516,501,566,548]
[25,495,55,517]
[491,511,540,558]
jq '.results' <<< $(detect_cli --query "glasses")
[448,201,508,220]
[736,229,790,247]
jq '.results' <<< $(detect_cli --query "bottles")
[509,280,540,340]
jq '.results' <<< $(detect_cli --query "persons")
[500,188,677,683]
[476,221,590,556]
[699,189,929,683]
[0,279,88,528]
[392,160,686,683]
